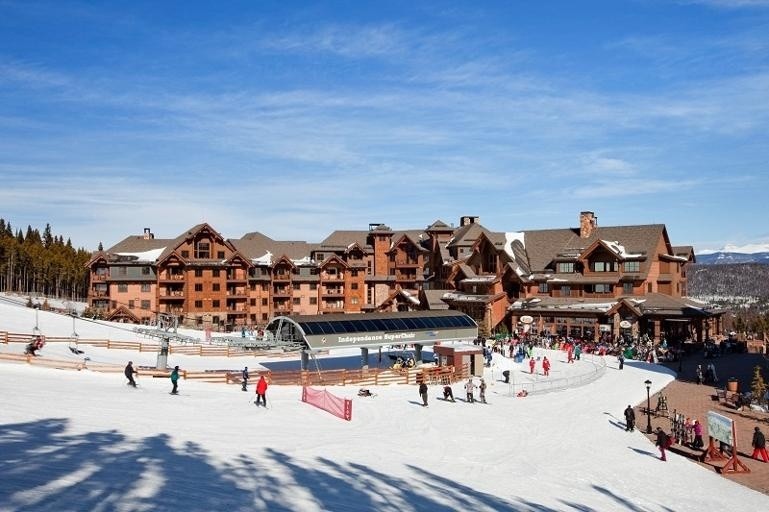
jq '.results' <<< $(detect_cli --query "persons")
[125,361,137,387]
[256,376,267,407]
[473,334,492,359]
[419,380,428,406]
[624,404,635,432]
[752,427,769,462]
[243,367,249,391]
[538,334,587,364]
[692,421,703,448]
[654,426,667,461]
[464,378,487,404]
[24,336,43,355]
[503,370,510,383]
[170,366,180,394]
[586,332,667,370]
[509,341,551,376]
[445,386,455,402]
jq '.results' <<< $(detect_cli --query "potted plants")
[728,376,739,391]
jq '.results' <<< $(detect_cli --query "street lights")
[644,379,655,436]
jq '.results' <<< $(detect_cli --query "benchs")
[667,441,703,461]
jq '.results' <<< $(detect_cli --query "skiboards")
[255,400,267,410]
[169,391,184,396]
[127,382,142,389]
[670,407,693,446]
